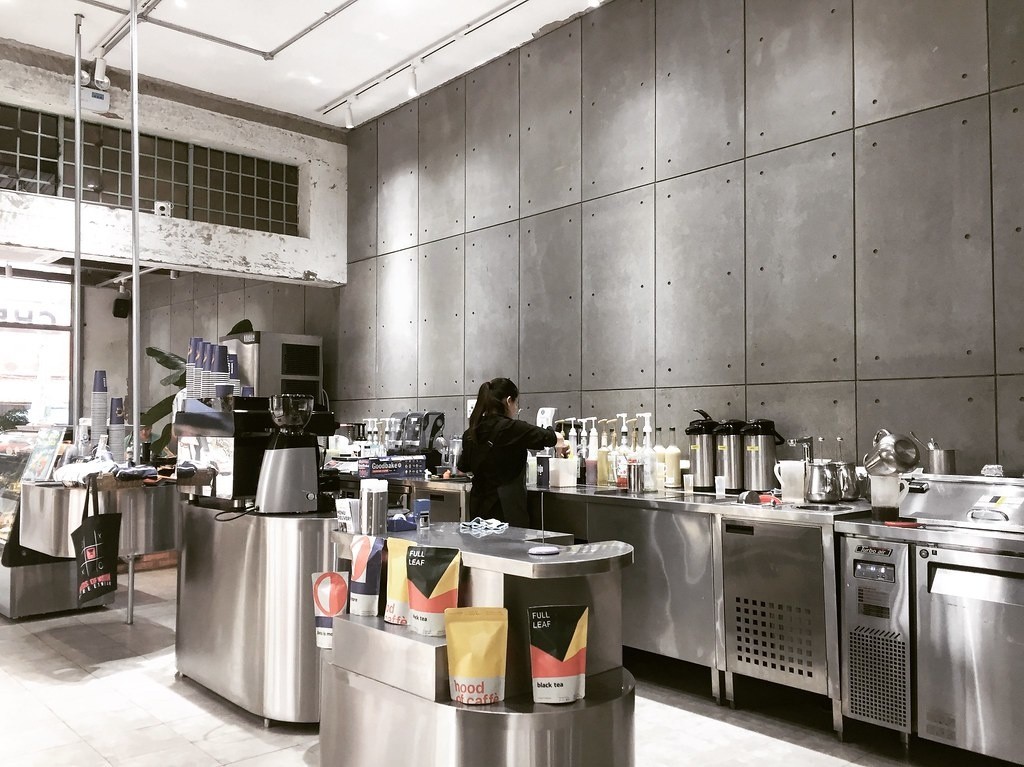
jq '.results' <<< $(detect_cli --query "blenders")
[387,410,446,475]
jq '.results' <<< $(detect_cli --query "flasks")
[684,407,785,493]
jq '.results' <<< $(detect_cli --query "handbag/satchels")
[70,473,122,609]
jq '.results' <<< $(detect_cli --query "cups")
[185,337,254,397]
[806,461,860,503]
[927,448,956,475]
[626,463,644,493]
[714,476,726,494]
[336,423,356,445]
[359,490,388,535]
[109,397,125,464]
[683,475,693,491]
[536,454,551,487]
[585,459,597,484]
[863,427,921,476]
[90,368,108,446]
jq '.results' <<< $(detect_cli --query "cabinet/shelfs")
[319,522,637,767]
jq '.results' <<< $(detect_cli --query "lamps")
[408,58,424,98]
[120,279,127,294]
[170,270,179,279]
[345,94,359,129]
[93,47,107,81]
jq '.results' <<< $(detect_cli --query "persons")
[457,378,569,528]
[126,413,167,457]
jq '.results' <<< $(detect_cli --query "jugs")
[869,474,909,521]
[774,459,805,503]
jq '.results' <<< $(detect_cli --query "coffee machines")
[251,392,336,514]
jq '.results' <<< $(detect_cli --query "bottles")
[652,426,666,488]
[597,445,609,485]
[665,426,681,487]
